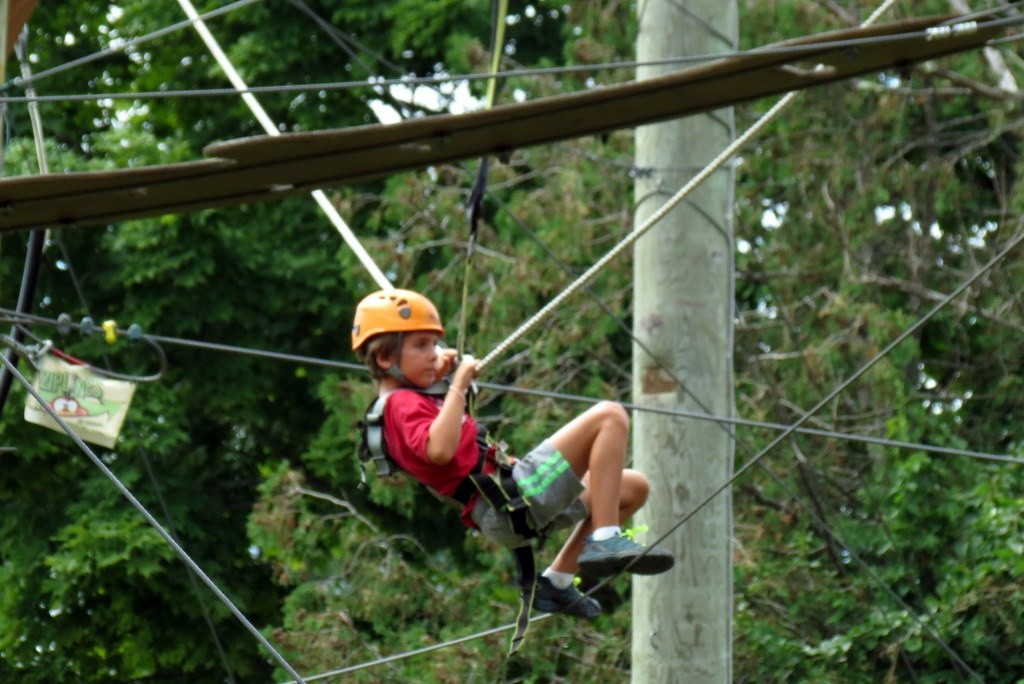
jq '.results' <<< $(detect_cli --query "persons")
[348,289,675,623]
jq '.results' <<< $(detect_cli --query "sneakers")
[525,572,601,619]
[577,524,675,575]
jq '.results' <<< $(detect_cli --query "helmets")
[351,288,444,351]
